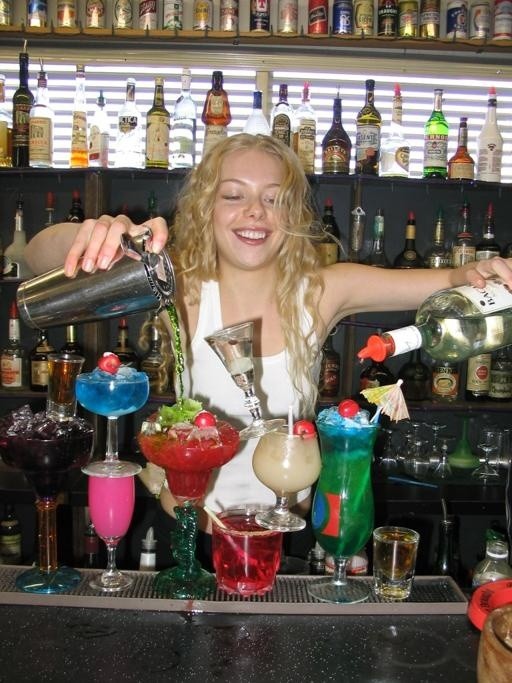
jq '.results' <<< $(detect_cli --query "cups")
[212,506,284,599]
[16,229,175,330]
[47,352,86,419]
[371,525,420,600]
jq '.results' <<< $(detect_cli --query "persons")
[23,132,512,577]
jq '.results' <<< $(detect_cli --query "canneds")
[28,0,48,28]
[163,0,183,31]
[58,0,78,29]
[250,0,270,32]
[278,0,512,41]
[220,0,239,32]
[113,0,134,29]
[0,0,13,26]
[86,0,104,28]
[192,0,213,31]
[139,0,158,30]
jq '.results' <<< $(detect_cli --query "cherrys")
[194,412,216,428]
[293,420,315,439]
[338,399,360,418]
[97,354,124,374]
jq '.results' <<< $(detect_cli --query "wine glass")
[74,371,149,478]
[0,414,98,596]
[378,417,511,484]
[307,416,375,605]
[137,420,241,601]
[253,425,322,531]
[204,321,288,441]
[87,474,135,591]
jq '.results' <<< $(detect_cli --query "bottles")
[1,495,25,569]
[477,85,503,185]
[471,540,512,592]
[321,97,352,176]
[82,518,102,572]
[1,301,174,401]
[382,84,411,180]
[356,327,511,405]
[172,68,198,168]
[87,89,110,168]
[296,81,318,176]
[0,74,13,168]
[431,496,461,587]
[314,179,512,271]
[70,64,91,168]
[355,79,381,178]
[0,0,512,44]
[271,83,294,149]
[147,77,171,168]
[12,52,35,170]
[3,186,158,280]
[423,89,449,181]
[243,91,270,137]
[137,524,160,573]
[355,273,512,361]
[117,78,142,169]
[203,70,233,158]
[447,116,475,180]
[28,71,53,169]
